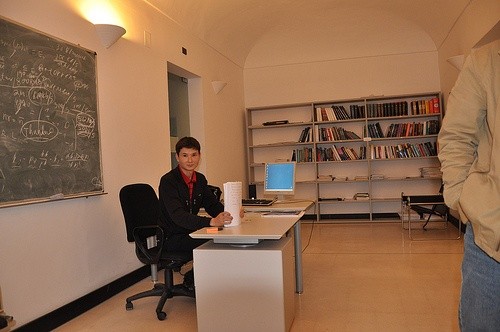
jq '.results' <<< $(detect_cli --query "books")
[291,98,440,200]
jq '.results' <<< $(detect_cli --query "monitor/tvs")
[264,162,295,202]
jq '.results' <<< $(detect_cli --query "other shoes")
[182,279,194,290]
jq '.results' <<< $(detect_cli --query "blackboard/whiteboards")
[0,15,108,208]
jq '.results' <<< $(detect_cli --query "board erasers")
[50,193,64,199]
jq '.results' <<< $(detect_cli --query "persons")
[436,39,500,332]
[158,136,244,287]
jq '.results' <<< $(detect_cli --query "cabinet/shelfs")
[245,91,443,223]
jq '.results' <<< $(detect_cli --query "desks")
[242,198,316,214]
[189,211,305,332]
[400,191,461,240]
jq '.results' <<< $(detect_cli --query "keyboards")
[242,199,274,205]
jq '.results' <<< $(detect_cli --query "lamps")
[446,54,463,71]
[209,81,228,94]
[94,23,126,48]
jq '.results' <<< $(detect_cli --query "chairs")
[410,183,448,228]
[119,183,195,320]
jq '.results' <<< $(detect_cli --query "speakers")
[249,184,256,199]
